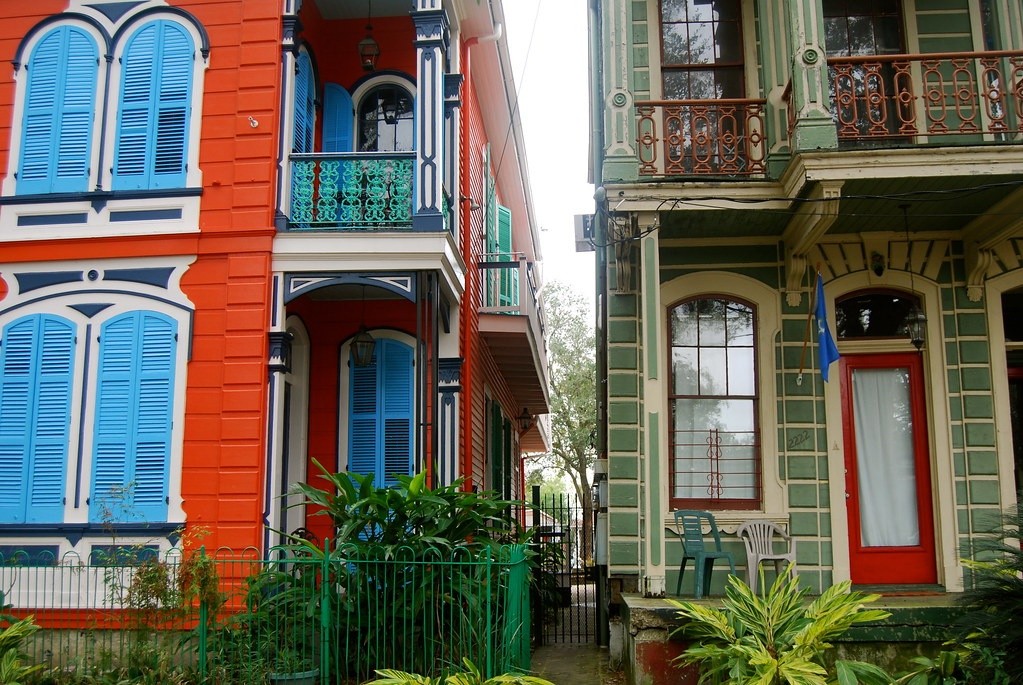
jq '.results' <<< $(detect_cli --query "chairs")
[737,520,800,596]
[674,510,738,600]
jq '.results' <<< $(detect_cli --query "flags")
[815,271,840,384]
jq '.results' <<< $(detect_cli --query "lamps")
[381,95,401,124]
[518,408,531,429]
[358,0,380,69]
[897,203,927,354]
[350,284,376,365]
